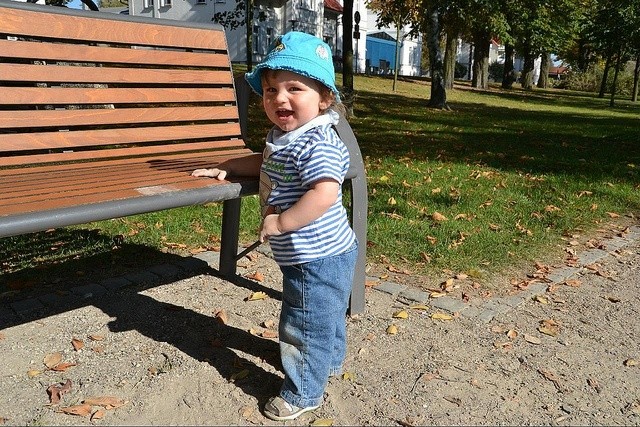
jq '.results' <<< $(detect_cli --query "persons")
[190,31,359,420]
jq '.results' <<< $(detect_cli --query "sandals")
[264,395,320,421]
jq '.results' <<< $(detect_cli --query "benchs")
[0,0,371,317]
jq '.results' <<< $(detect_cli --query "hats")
[244,31,339,102]
